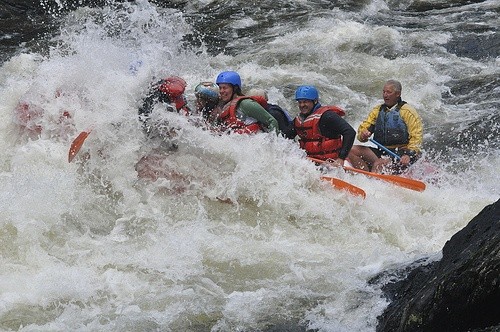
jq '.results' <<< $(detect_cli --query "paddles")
[319,177,366,200]
[304,157,426,194]
[367,136,413,168]
[66,120,99,162]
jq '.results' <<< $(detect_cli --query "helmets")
[216,71,241,88]
[194,81,219,105]
[245,89,269,101]
[294,85,318,101]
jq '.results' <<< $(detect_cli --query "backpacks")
[266,103,296,139]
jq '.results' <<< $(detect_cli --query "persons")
[347,80,424,176]
[139,76,192,117]
[285,85,355,171]
[194,71,297,144]
